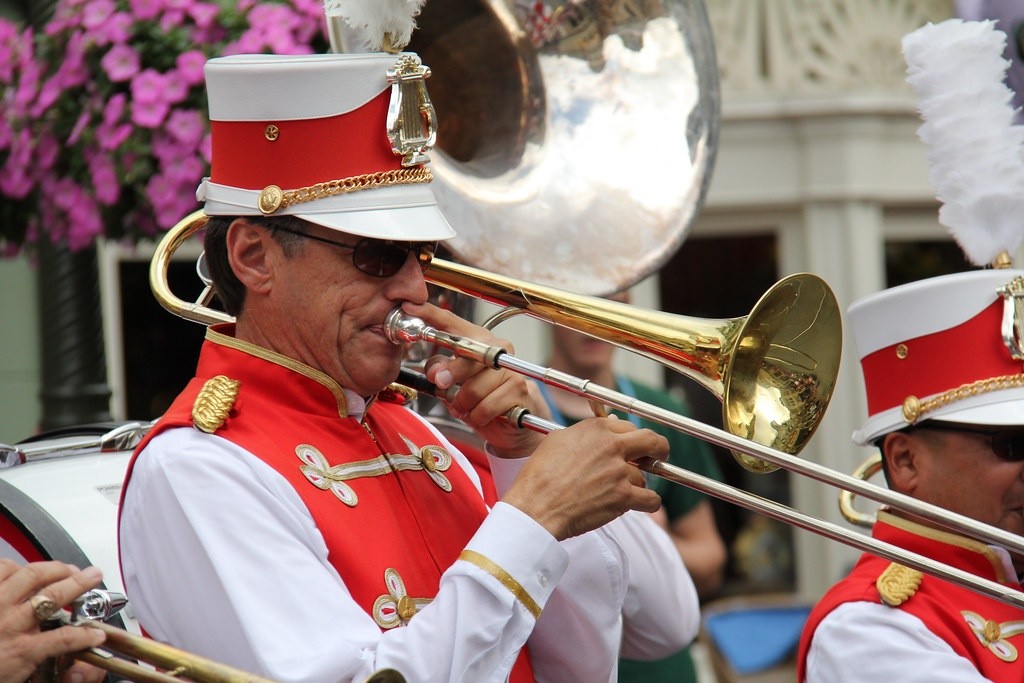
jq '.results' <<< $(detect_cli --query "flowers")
[0,0,331,271]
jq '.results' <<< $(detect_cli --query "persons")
[118,53,671,683]
[530,288,723,683]
[797,270,1024,683]
[0,558,110,683]
[527,379,703,659]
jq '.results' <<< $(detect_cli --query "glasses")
[915,425,1024,462]
[276,227,438,277]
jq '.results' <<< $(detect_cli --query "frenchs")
[319,0,725,407]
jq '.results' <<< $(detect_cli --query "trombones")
[150,208,1024,627]
[33,589,406,682]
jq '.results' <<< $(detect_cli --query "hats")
[196,0,458,241]
[846,18,1024,445]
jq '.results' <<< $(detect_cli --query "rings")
[29,595,59,620]
